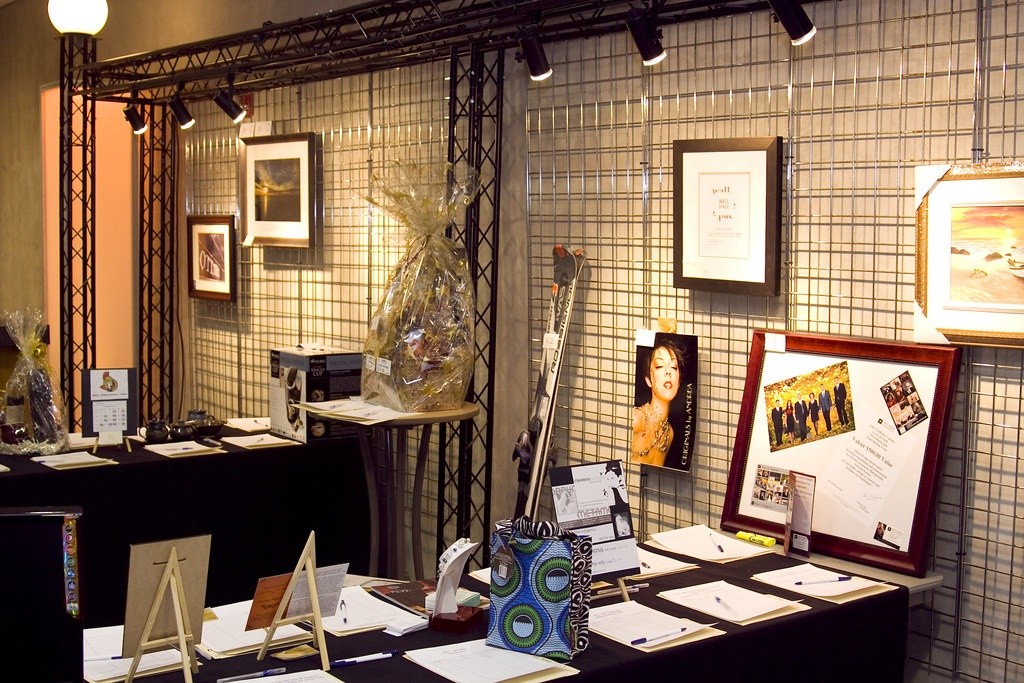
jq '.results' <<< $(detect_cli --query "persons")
[771,376,849,447]
[880,370,925,428]
[631,337,690,466]
[754,469,790,503]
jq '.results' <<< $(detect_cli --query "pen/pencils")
[709,532,724,553]
[84,655,123,662]
[640,560,651,569]
[629,626,686,645]
[795,577,852,586]
[165,447,193,451]
[716,596,735,613]
[331,648,405,666]
[254,420,268,427]
[341,600,348,625]
[312,348,324,350]
[330,403,343,409]
[257,438,264,443]
[37,460,65,463]
[217,668,286,683]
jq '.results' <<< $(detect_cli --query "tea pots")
[169,420,196,439]
[139,420,168,443]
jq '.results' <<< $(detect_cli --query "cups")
[288,399,296,420]
[312,426,323,432]
[188,409,215,421]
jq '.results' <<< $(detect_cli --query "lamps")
[511,30,555,84]
[768,0,819,47]
[47,0,109,38]
[123,91,149,136]
[624,6,670,67]
[168,82,196,131]
[211,74,248,124]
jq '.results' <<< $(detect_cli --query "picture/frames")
[238,132,316,251]
[913,160,1024,348]
[716,329,963,579]
[187,213,235,303]
[673,135,783,298]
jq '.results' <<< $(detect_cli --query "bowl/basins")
[192,420,227,435]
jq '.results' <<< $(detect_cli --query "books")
[370,580,457,620]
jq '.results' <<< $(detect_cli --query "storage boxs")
[268,343,364,447]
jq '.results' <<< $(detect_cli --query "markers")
[736,530,778,546]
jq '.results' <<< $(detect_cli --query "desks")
[80,530,944,683]
[0,423,393,631]
[360,400,481,583]
